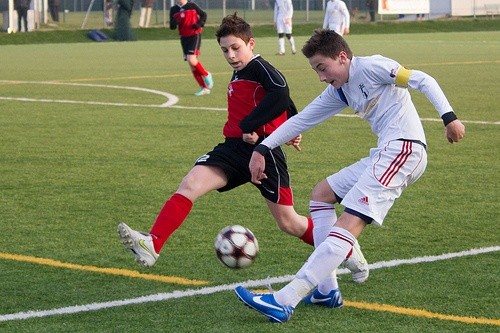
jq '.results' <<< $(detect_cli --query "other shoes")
[292,47,298,54]
[278,50,286,55]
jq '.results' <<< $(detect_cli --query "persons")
[322,0,350,36]
[415,14,426,22]
[273,0,296,55]
[234,31,465,321]
[168,0,213,96]
[10,0,157,33]
[117,12,369,283]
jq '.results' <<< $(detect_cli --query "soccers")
[214,224,260,269]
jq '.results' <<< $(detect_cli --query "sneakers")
[194,71,214,96]
[117,222,160,269]
[233,285,296,324]
[342,238,369,283]
[303,288,344,308]
